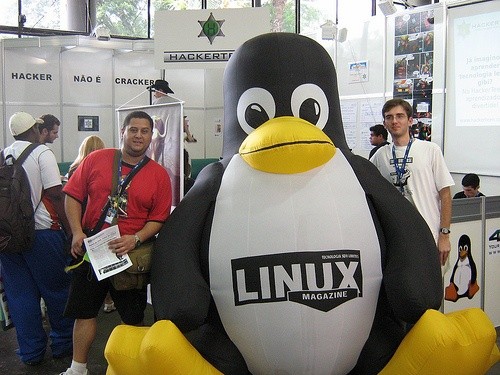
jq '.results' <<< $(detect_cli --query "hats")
[146,80,174,94]
[9,112,44,137]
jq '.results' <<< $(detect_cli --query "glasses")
[385,115,405,122]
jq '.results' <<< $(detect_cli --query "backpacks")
[0,142,48,258]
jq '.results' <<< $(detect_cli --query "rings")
[76,251,78,254]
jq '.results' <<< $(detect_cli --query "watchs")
[134,233,143,248]
[440,227,451,234]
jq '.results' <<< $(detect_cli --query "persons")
[66,135,121,312]
[0,111,73,367]
[36,113,61,145]
[369,124,390,160]
[146,79,197,177]
[452,174,487,199]
[60,111,173,375]
[369,99,455,266]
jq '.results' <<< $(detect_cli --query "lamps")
[91,24,111,41]
[377,0,418,16]
[319,19,337,40]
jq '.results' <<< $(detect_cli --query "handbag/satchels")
[109,235,157,291]
[63,228,94,286]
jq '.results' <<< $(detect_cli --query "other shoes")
[59,368,90,375]
[103,300,116,312]
